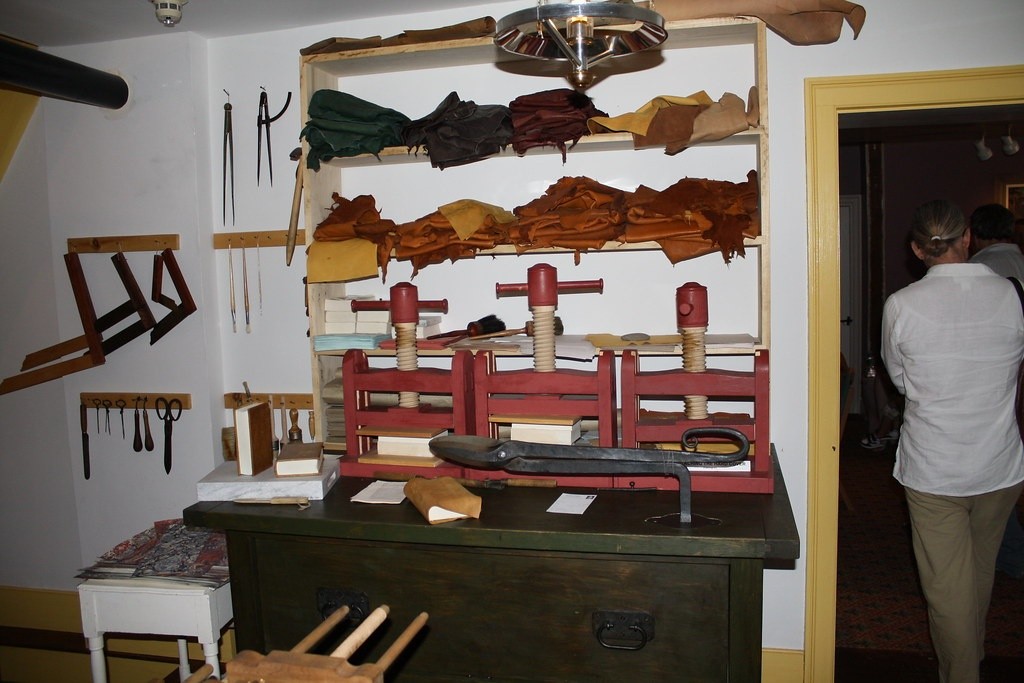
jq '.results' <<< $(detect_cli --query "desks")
[77,519,233,683]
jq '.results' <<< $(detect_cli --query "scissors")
[155,396,183,475]
[427,427,749,526]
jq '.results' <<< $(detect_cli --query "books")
[403,476,482,524]
[274,442,324,478]
[233,401,273,476]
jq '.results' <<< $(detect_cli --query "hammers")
[286,147,304,267]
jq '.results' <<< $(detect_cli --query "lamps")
[494,0,669,88]
[973,129,993,161]
[1001,123,1021,156]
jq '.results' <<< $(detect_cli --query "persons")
[969,204,1024,282]
[880,200,1024,683]
[857,384,905,448]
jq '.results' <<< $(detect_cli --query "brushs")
[280,395,289,452]
[289,408,302,445]
[268,395,280,460]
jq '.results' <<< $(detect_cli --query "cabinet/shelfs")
[299,15,769,452]
[184,443,800,683]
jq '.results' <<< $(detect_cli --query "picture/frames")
[1006,184,1024,219]
